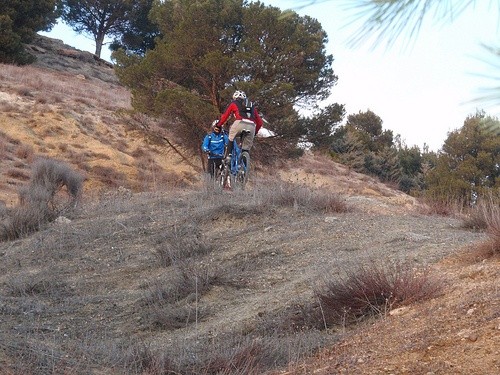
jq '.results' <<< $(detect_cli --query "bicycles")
[207,127,252,191]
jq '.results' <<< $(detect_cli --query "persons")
[215,88,264,169]
[201,119,230,180]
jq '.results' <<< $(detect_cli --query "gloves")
[206,150,211,156]
[214,123,222,129]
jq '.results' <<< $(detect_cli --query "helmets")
[232,90,247,101]
[212,119,224,130]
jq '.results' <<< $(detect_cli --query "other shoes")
[225,153,232,161]
